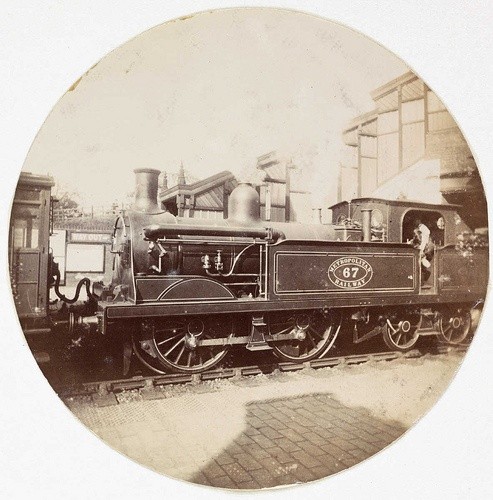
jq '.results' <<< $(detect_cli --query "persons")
[407,218,436,269]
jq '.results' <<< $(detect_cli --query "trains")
[7,168,488,377]
